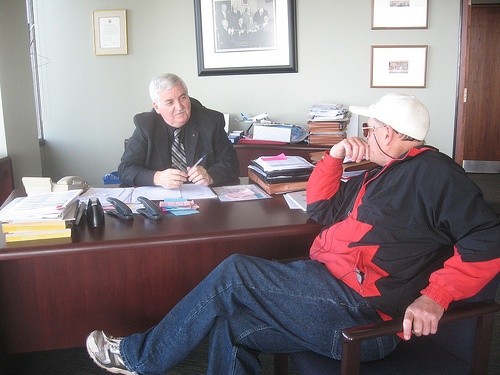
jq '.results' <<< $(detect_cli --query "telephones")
[52,176,88,191]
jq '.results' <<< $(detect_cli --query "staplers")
[107,197,134,220]
[137,196,162,220]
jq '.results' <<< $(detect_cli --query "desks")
[0,184,325,357]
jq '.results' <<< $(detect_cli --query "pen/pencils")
[192,155,206,168]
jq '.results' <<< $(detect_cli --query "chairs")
[271,267,500,375]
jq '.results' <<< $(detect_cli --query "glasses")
[361,123,381,138]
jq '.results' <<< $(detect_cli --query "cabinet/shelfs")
[233,143,331,177]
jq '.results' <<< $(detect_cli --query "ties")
[171,130,188,176]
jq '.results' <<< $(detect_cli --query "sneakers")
[86,330,140,375]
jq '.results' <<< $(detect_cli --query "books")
[247,156,316,194]
[308,104,352,145]
[0,189,84,242]
[310,151,379,183]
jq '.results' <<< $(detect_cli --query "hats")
[349,94,430,142]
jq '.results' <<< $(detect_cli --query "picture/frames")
[92,9,127,55]
[371,45,428,89]
[194,0,298,76]
[371,0,429,31]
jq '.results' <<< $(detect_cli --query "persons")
[118,73,240,188]
[86,92,500,375]
[215,4,269,49]
